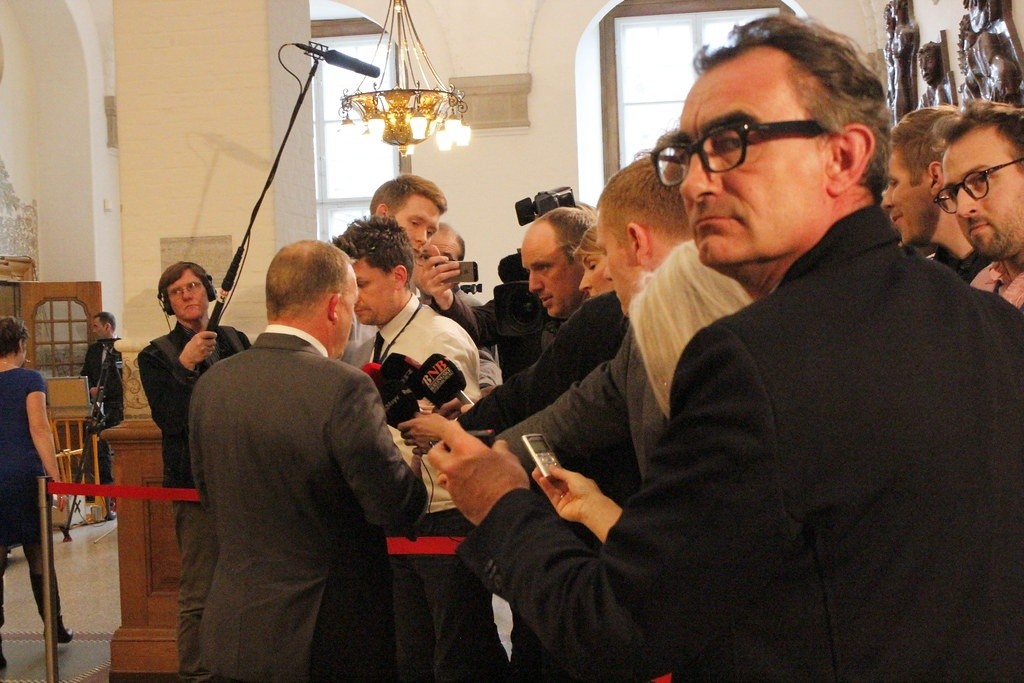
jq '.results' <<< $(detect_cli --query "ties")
[372,331,385,364]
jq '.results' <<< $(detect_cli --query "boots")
[0,584,7,670]
[30,573,73,644]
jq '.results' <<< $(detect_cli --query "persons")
[915,42,947,108]
[0,316,73,671]
[81,312,122,510]
[883,99,1024,312]
[189,152,755,683]
[425,18,1024,683]
[137,262,253,683]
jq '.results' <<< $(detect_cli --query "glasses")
[168,282,204,301]
[651,119,828,187]
[933,158,1024,214]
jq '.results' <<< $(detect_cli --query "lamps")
[341,0,471,157]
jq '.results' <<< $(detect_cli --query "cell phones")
[440,261,479,282]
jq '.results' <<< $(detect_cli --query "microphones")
[361,353,476,432]
[294,44,381,78]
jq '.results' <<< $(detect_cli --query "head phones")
[157,272,217,316]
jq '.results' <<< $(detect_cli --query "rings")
[60,496,64,498]
